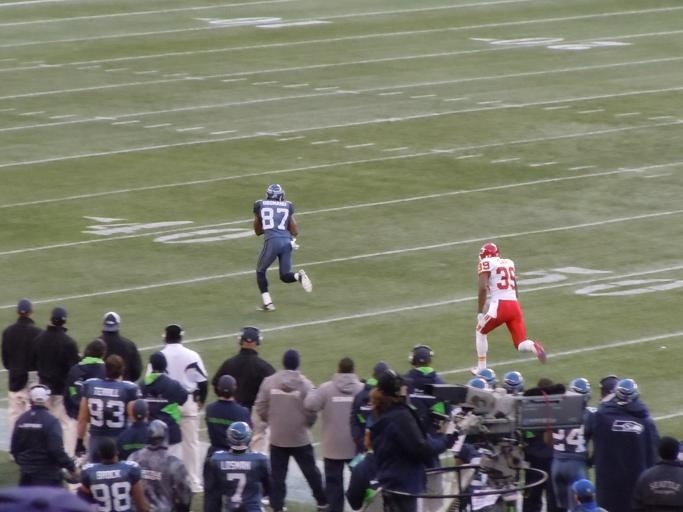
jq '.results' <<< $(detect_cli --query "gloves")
[476,312,487,327]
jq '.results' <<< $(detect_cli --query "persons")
[253,184,312,311]
[471,242,546,378]
[1,300,209,512]
[203,326,683,512]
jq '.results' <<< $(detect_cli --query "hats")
[147,420,170,448]
[160,321,186,340]
[133,399,149,420]
[151,351,167,370]
[242,326,260,342]
[17,299,32,315]
[103,311,120,333]
[28,385,51,403]
[52,308,67,323]
[217,374,238,395]
[281,348,303,366]
[409,345,432,366]
[571,479,596,497]
[377,369,410,396]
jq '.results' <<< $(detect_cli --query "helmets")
[267,183,285,201]
[615,378,640,401]
[599,374,622,393]
[571,377,591,394]
[478,368,497,386]
[479,243,499,260]
[226,421,253,451]
[468,378,488,390]
[503,371,524,393]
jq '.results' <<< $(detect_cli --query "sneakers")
[298,269,312,293]
[256,301,276,312]
[191,481,205,493]
[471,367,481,377]
[533,341,546,364]
